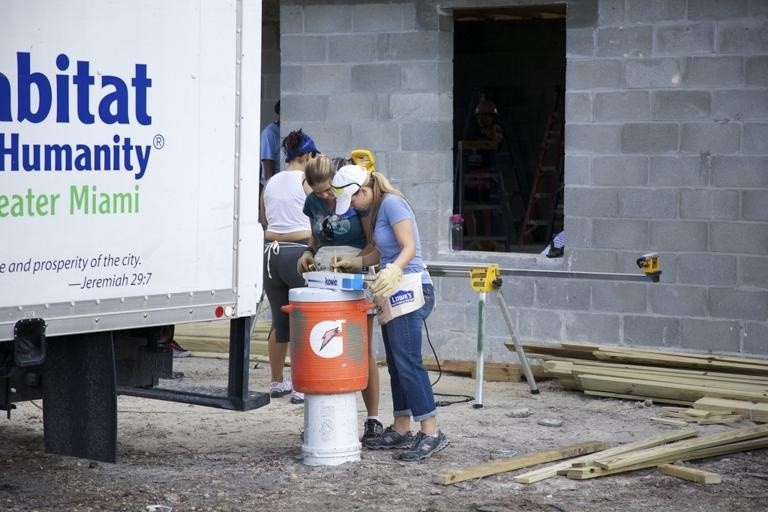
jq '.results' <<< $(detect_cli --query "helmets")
[474,100,499,116]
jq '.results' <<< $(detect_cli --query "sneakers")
[269,380,294,398]
[392,429,450,461]
[172,339,192,359]
[362,419,385,443]
[363,425,418,450]
[290,389,305,404]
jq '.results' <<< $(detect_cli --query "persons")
[296,153,382,442]
[259,100,280,191]
[258,129,321,404]
[332,165,449,462]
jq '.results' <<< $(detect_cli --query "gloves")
[329,255,364,273]
[296,250,321,276]
[363,262,405,298]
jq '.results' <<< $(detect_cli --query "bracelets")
[303,246,316,257]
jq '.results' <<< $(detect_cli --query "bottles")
[449,214,465,252]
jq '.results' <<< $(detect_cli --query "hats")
[282,130,322,162]
[329,164,370,216]
